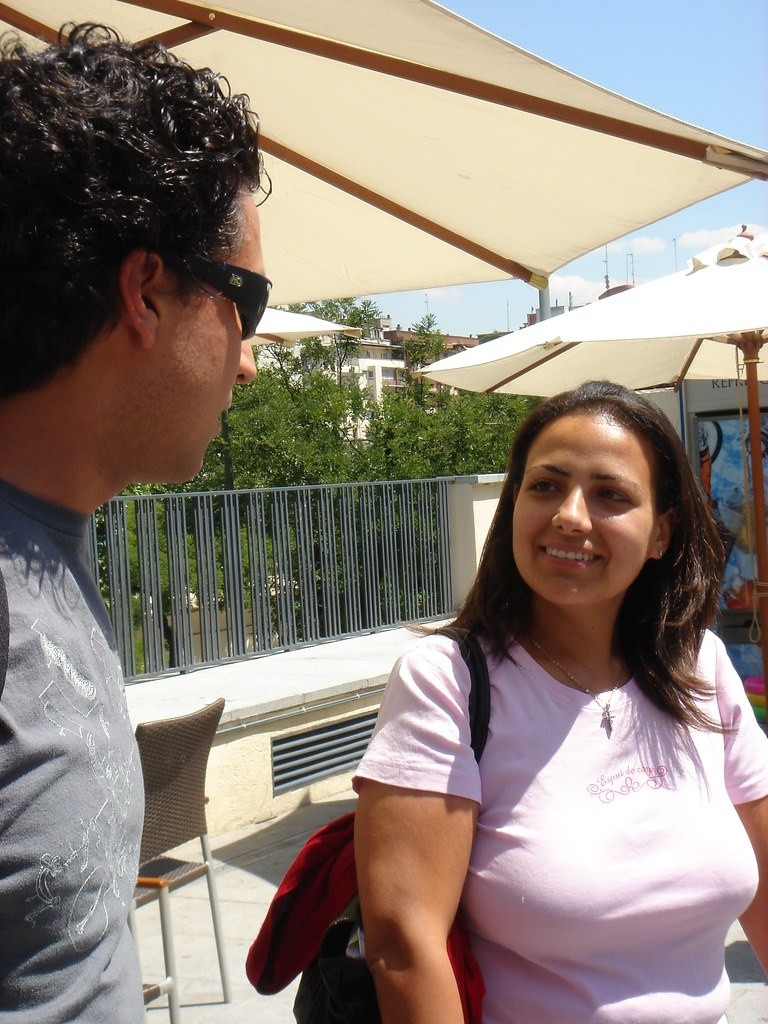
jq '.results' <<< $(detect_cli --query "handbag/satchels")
[292,628,491,1024]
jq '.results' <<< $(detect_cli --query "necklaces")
[530,639,626,732]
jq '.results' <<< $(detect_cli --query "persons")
[0,20,275,1024]
[352,381,768,1024]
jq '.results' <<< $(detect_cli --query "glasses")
[198,261,273,341]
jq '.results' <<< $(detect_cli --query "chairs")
[114,698,230,1023]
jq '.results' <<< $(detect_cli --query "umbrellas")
[247,307,363,345]
[411,223,768,668]
[0,0,768,307]
